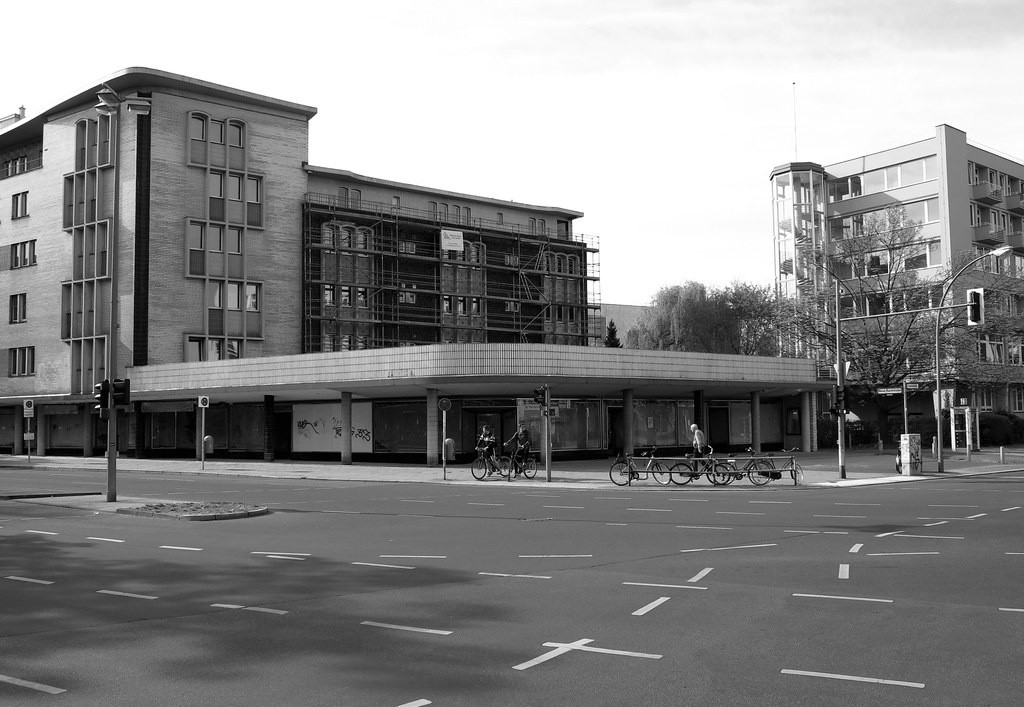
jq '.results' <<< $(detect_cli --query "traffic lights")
[533,386,546,406]
[833,386,844,407]
[93,379,109,411]
[113,378,131,405]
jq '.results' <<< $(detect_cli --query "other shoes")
[514,472,518,478]
[522,458,527,464]
[496,469,502,475]
[486,471,492,477]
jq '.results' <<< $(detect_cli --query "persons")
[476,425,497,476]
[690,423,710,479]
[503,423,532,479]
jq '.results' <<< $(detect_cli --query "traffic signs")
[877,384,918,394]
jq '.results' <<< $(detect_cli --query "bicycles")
[503,443,537,482]
[471,447,513,480]
[609,445,805,486]
[895,440,903,475]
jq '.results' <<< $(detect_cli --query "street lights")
[95,81,153,501]
[935,245,1015,473]
